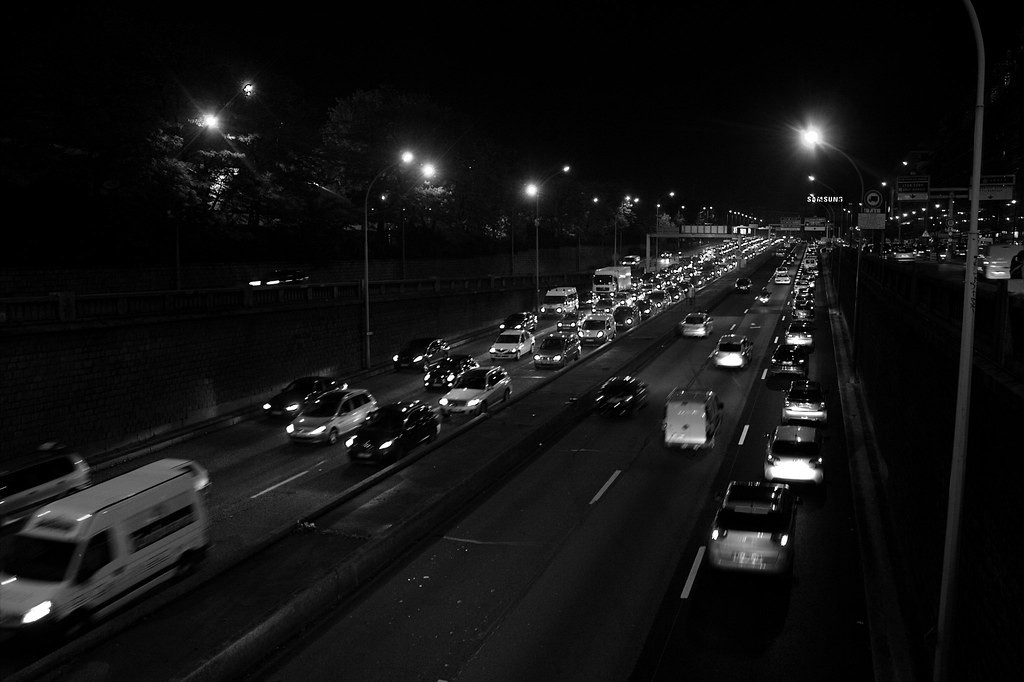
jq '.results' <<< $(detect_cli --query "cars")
[774,248,797,285]
[680,313,715,338]
[148,459,211,505]
[855,230,1024,280]
[734,277,753,296]
[488,235,776,367]
[595,375,651,418]
[783,242,820,347]
[714,334,754,370]
[259,336,513,470]
[769,344,810,378]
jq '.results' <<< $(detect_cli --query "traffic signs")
[969,175,1015,201]
[896,175,929,201]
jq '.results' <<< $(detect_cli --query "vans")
[0,465,212,652]
[662,387,726,450]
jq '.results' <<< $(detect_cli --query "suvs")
[779,378,828,423]
[763,423,826,488]
[704,481,801,576]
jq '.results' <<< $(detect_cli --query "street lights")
[882,181,1016,245]
[510,165,764,309]
[363,149,413,368]
[799,125,865,340]
[808,175,844,311]
[173,112,221,284]
